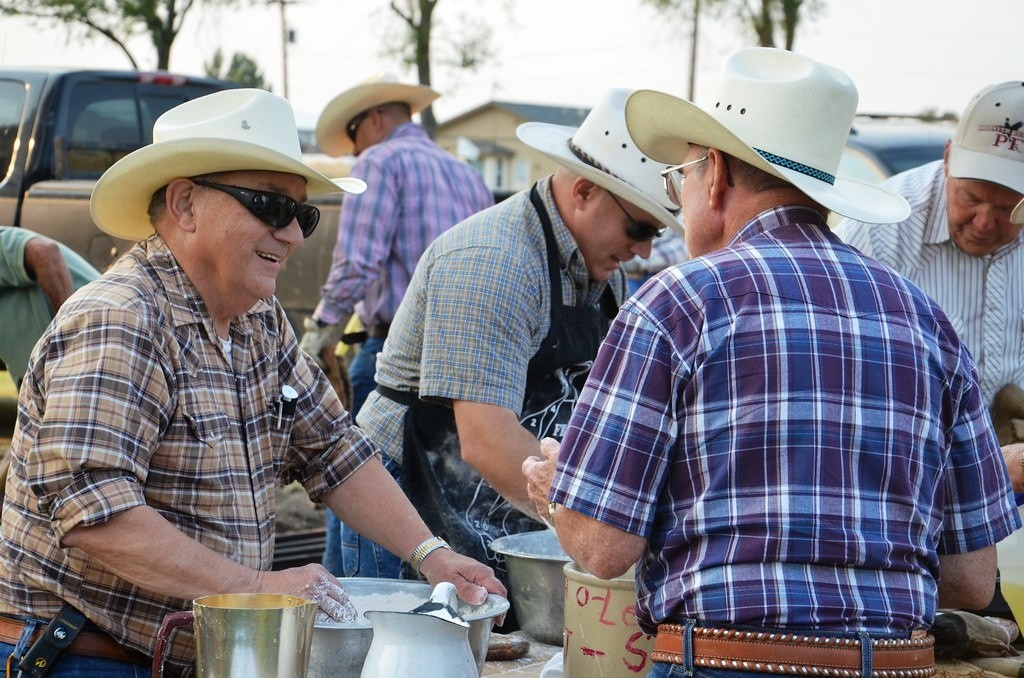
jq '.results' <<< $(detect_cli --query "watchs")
[548,502,558,527]
[410,536,454,577]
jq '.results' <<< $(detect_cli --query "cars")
[837,122,956,186]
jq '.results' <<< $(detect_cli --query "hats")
[316,84,440,156]
[625,45,911,226]
[948,82,1024,196]
[515,96,685,237]
[90,88,368,241]
[1009,197,1024,224]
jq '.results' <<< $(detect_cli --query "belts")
[0,616,152,664]
[650,624,936,678]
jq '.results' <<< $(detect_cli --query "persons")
[323,88,682,580]
[299,72,496,429]
[0,88,507,678]
[0,226,100,396]
[522,46,1024,678]
[831,81,1024,508]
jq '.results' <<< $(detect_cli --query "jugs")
[152,595,317,677]
[359,581,479,678]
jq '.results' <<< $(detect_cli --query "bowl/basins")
[490,530,575,648]
[308,576,509,678]
[562,561,653,677]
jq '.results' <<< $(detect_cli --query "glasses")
[348,109,384,141]
[661,155,734,208]
[606,190,663,242]
[193,179,321,239]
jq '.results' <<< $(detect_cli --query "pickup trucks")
[1,64,343,339]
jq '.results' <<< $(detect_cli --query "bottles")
[993,492,1024,638]
